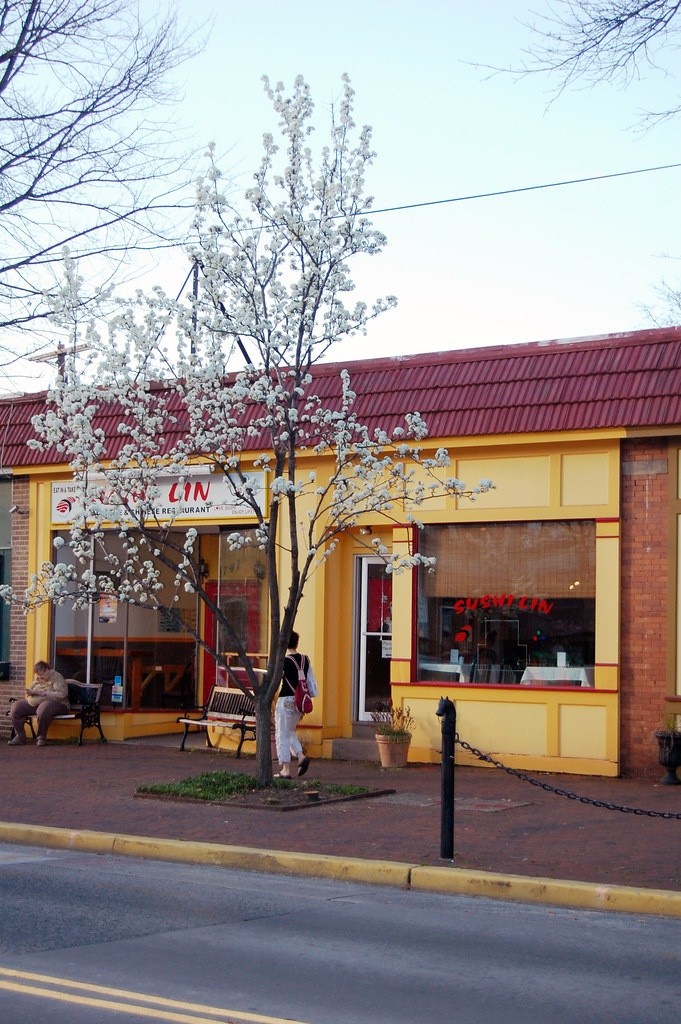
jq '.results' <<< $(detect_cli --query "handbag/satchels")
[306,663,318,697]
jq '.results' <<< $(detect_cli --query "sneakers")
[36,735,46,746]
[7,735,26,745]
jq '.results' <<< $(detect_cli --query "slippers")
[297,756,310,776]
[273,771,292,779]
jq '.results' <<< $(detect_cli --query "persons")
[273,629,312,781]
[7,659,72,748]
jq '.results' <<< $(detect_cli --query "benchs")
[6,678,108,748]
[175,684,274,760]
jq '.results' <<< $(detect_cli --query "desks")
[520,663,596,687]
[57,633,195,708]
[418,658,514,682]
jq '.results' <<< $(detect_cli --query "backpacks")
[284,654,313,715]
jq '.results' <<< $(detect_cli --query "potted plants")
[369,696,413,767]
